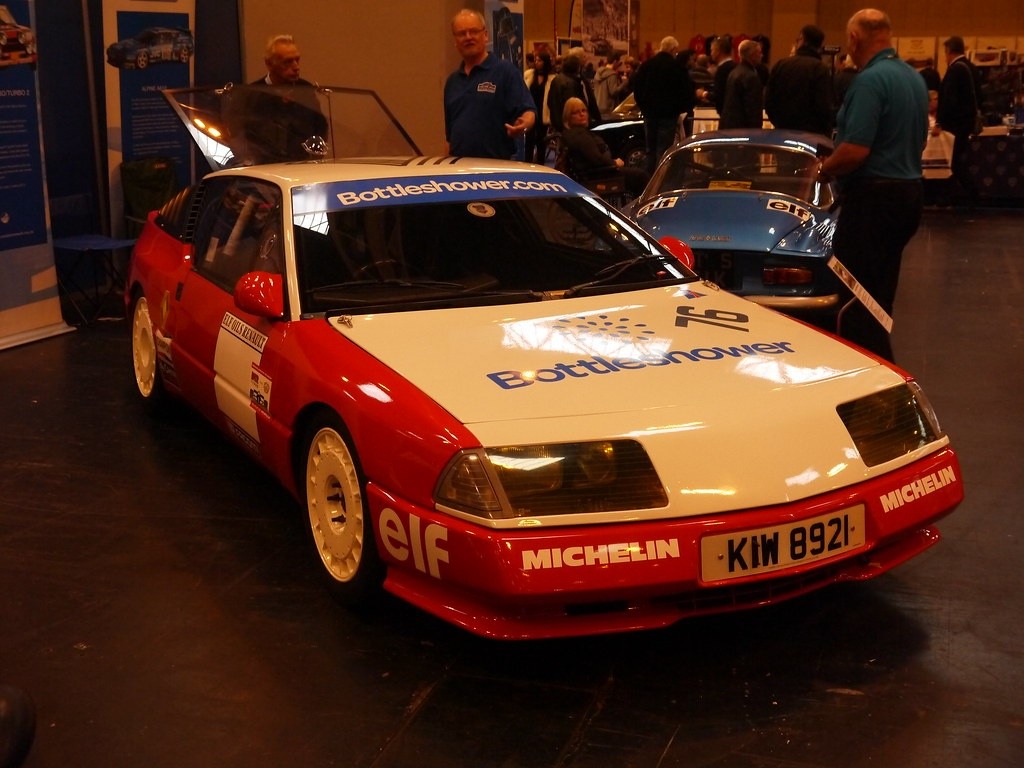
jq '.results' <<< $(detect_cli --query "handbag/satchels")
[954,62,984,136]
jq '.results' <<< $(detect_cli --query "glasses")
[454,27,486,38]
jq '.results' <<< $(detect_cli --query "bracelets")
[701,90,708,99]
[818,163,827,179]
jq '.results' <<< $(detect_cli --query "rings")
[524,128,528,132]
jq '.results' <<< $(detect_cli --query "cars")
[124,85,964,640]
[620,130,841,308]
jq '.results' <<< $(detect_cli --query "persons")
[523,7,985,365]
[245,34,330,164]
[444,8,538,163]
[491,7,523,71]
